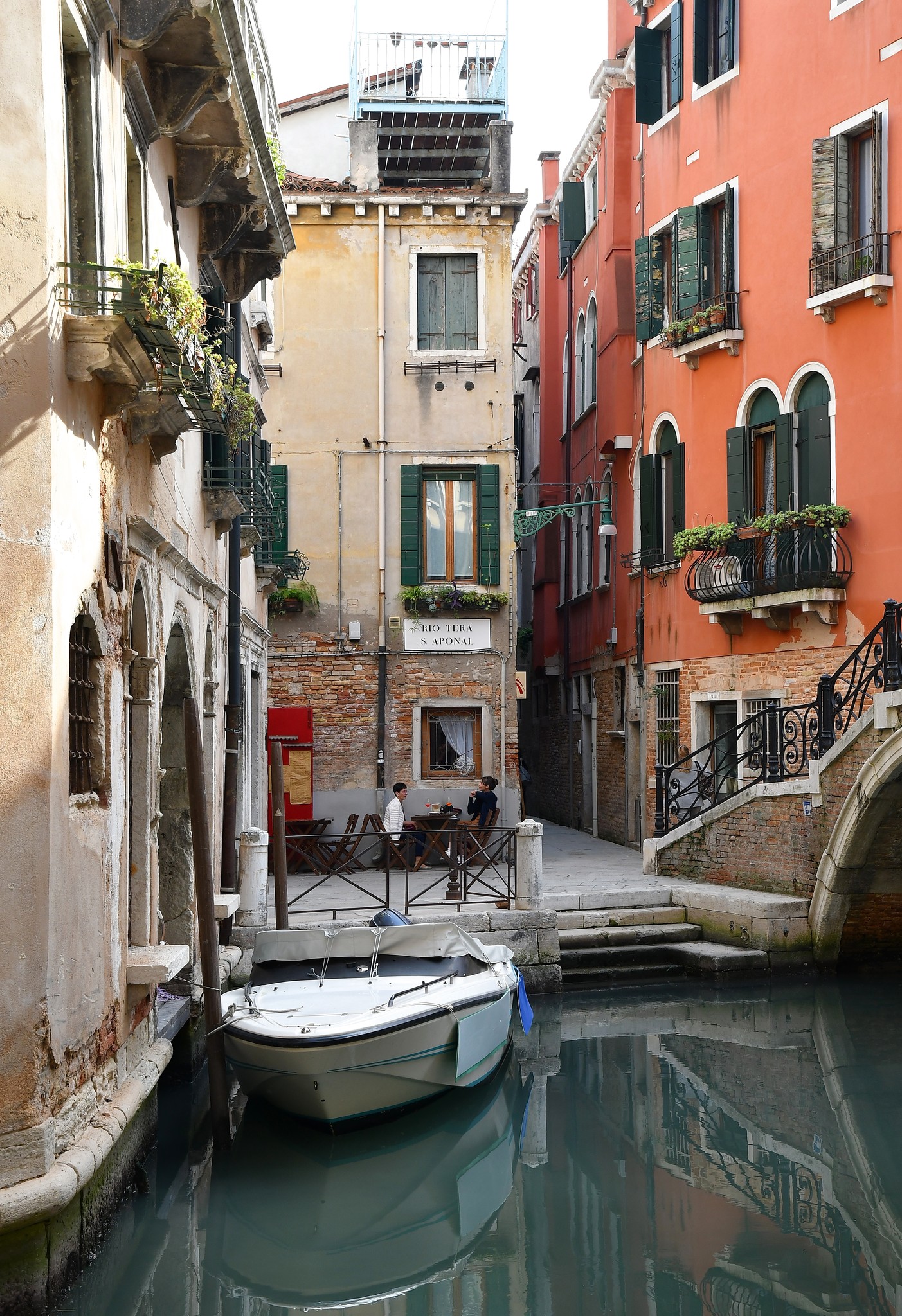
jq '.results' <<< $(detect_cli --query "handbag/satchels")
[404,824,416,831]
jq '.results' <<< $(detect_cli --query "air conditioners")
[695,558,741,597]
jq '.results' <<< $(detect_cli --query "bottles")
[425,799,431,815]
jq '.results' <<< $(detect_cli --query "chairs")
[313,807,500,876]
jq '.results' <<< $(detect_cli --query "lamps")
[514,494,617,542]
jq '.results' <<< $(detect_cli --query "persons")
[440,776,498,864]
[379,782,432,869]
[662,745,713,823]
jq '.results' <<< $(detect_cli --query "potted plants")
[658,304,727,343]
[673,502,852,562]
[856,255,873,276]
[87,250,259,456]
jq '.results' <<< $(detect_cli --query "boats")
[210,1038,551,1309]
[220,906,536,1137]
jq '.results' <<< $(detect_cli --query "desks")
[285,817,334,875]
[410,814,457,872]
[410,814,458,850]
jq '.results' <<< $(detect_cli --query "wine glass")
[446,797,453,814]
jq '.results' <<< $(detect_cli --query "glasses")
[402,791,409,794]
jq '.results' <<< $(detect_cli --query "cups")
[431,803,440,812]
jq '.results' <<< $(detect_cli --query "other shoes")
[457,855,464,864]
[414,864,432,869]
[440,850,451,860]
